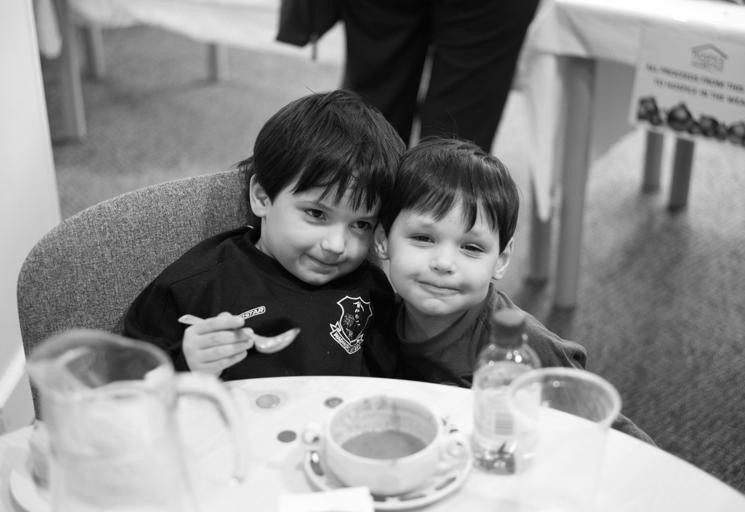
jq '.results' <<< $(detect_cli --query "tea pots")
[23,325,250,511]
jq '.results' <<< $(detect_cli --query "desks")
[69,0,347,83]
[514,0,745,315]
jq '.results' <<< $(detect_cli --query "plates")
[7,449,53,511]
[306,426,475,510]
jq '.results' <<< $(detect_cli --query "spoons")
[178,313,301,354]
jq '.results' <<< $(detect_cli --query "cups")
[323,397,445,495]
[501,363,624,511]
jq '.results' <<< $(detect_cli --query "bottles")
[467,306,543,477]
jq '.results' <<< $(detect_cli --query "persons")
[365,134,660,450]
[104,87,397,379]
[274,1,544,158]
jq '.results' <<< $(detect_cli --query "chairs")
[14,169,252,414]
